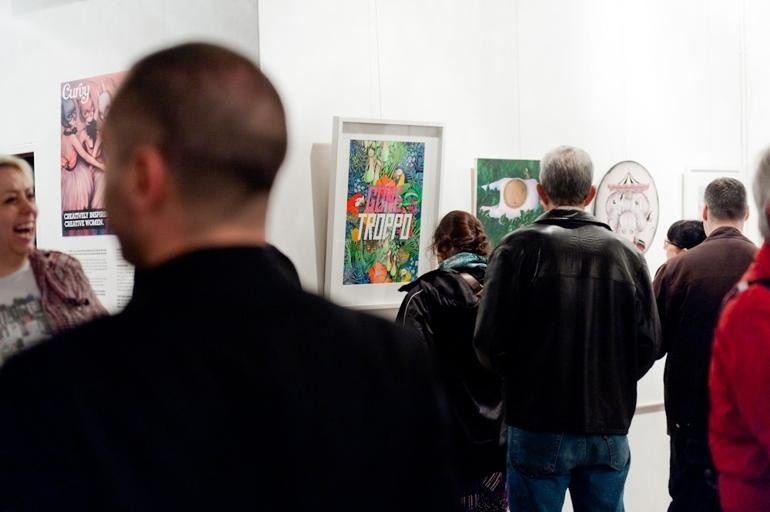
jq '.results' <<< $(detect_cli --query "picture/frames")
[323,115,448,312]
[683,165,743,220]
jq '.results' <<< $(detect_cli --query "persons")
[1,41,461,510]
[651,177,759,512]
[361,145,405,188]
[707,146,770,512]
[664,220,704,261]
[62,92,112,235]
[395,210,509,512]
[0,153,109,369]
[473,146,663,512]
